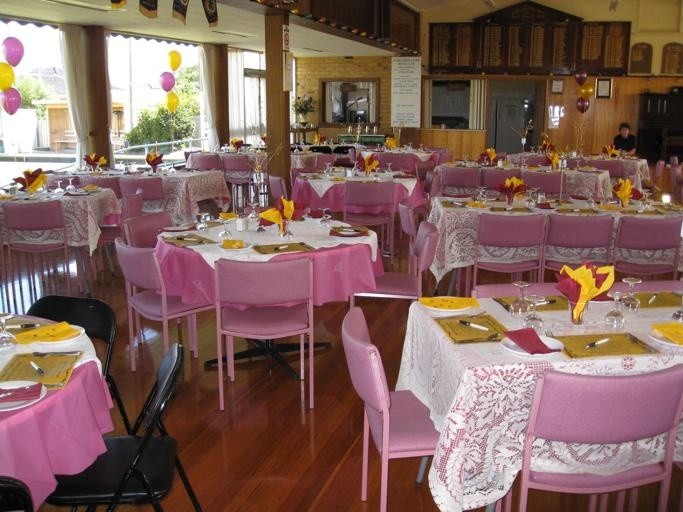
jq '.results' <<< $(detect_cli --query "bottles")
[8,182,18,198]
[237,210,244,232]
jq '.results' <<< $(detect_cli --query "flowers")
[291,93,315,116]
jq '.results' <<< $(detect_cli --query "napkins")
[650,320,682,343]
[556,257,618,323]
[419,296,480,310]
[3,380,42,402]
[504,328,561,355]
[11,321,78,341]
[260,195,310,232]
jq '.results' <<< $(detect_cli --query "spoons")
[34,350,83,358]
[456,333,500,345]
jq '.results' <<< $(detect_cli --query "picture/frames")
[595,77,613,100]
[550,78,565,94]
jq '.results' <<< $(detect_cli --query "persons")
[613,122,636,155]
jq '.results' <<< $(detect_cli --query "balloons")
[0,87,22,116]
[160,72,176,92]
[575,68,587,85]
[165,92,179,112]
[577,96,590,113]
[581,83,594,101]
[2,36,24,66]
[168,50,182,71]
[0,62,15,91]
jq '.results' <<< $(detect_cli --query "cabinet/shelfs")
[636,86,683,164]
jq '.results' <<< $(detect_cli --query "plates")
[647,326,682,349]
[570,196,588,204]
[0,381,49,411]
[33,325,90,344]
[500,336,565,359]
[67,190,88,195]
[335,228,363,238]
[592,297,614,304]
[217,240,253,251]
[466,203,490,211]
[423,294,472,312]
[595,205,612,215]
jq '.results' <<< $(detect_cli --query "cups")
[569,302,588,326]
[276,221,288,242]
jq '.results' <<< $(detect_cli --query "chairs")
[25,295,131,432]
[211,258,317,411]
[288,136,682,270]
[471,210,543,286]
[2,473,33,511]
[43,344,201,511]
[504,364,683,512]
[469,281,681,299]
[256,206,327,220]
[121,210,222,348]
[113,233,229,377]
[398,202,459,293]
[535,210,616,284]
[347,220,439,311]
[1,149,269,303]
[611,216,682,283]
[340,304,502,511]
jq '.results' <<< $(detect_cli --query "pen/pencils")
[504,208,513,211]
[0,323,41,330]
[29,361,44,377]
[529,299,556,307]
[451,201,463,206]
[635,210,644,213]
[584,336,610,349]
[175,237,198,241]
[341,228,359,232]
[457,319,489,331]
[571,209,581,212]
[648,295,657,305]
[272,245,288,251]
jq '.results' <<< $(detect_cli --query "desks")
[394,299,683,511]
[289,125,318,144]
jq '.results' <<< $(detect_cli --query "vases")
[298,113,306,123]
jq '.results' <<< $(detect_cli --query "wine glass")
[606,277,642,330]
[475,185,485,200]
[319,207,331,234]
[510,278,547,332]
[524,185,540,211]
[375,143,381,152]
[163,158,176,176]
[54,176,77,197]
[247,202,261,232]
[117,159,137,174]
[352,160,361,176]
[0,312,14,354]
[406,141,414,153]
[373,162,393,180]
[195,212,231,241]
[324,161,332,177]
